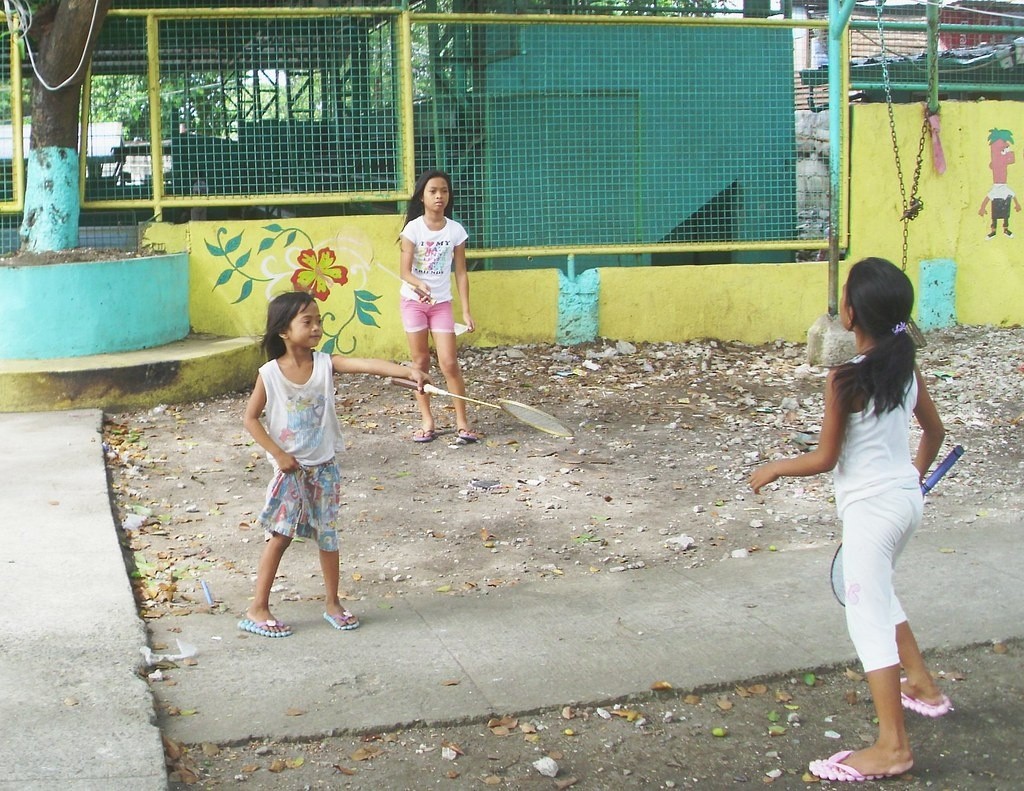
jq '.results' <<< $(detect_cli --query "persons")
[393,170,479,442]
[236,292,435,637]
[747,256,952,783]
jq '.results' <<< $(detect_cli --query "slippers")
[323,611,359,630]
[237,618,292,637]
[810,751,893,781]
[899,677,951,716]
[414,427,434,441]
[458,428,477,441]
